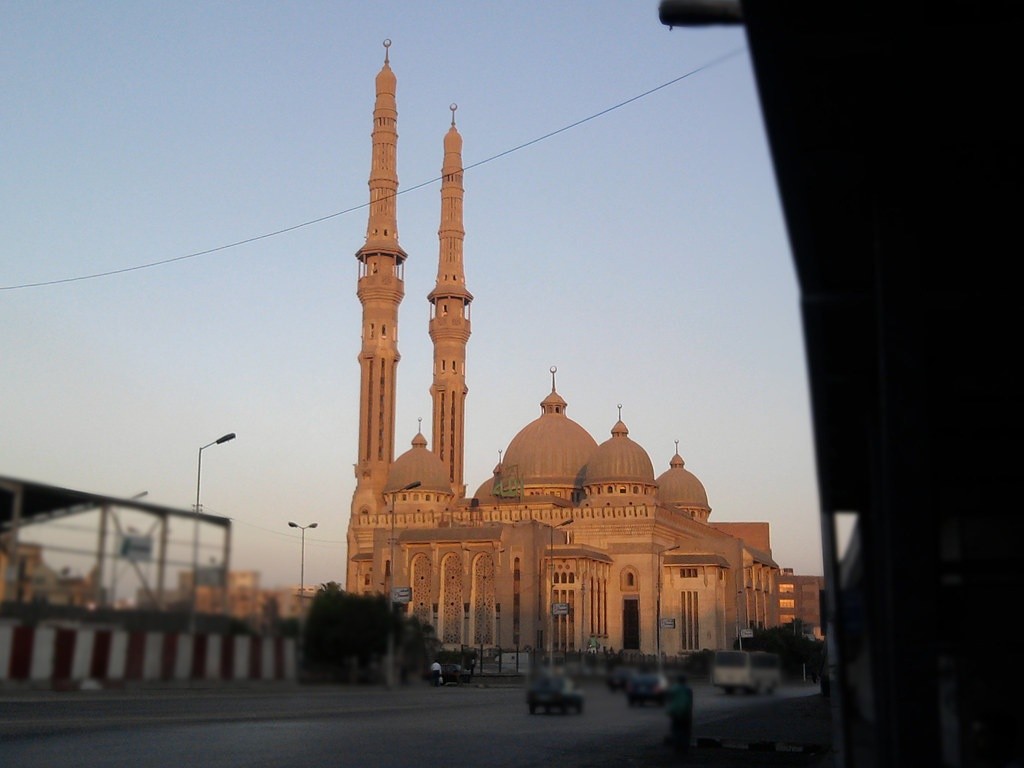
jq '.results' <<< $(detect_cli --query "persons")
[431,658,442,688]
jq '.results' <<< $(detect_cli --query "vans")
[711,651,780,695]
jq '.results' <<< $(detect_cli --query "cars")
[527,664,591,716]
[432,650,476,686]
[628,665,692,710]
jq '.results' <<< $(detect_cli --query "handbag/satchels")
[439,676,443,683]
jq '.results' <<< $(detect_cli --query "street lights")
[288,522,318,597]
[194,432,236,604]
[388,481,422,685]
[656,546,680,659]
[735,589,744,653]
[552,520,573,667]
